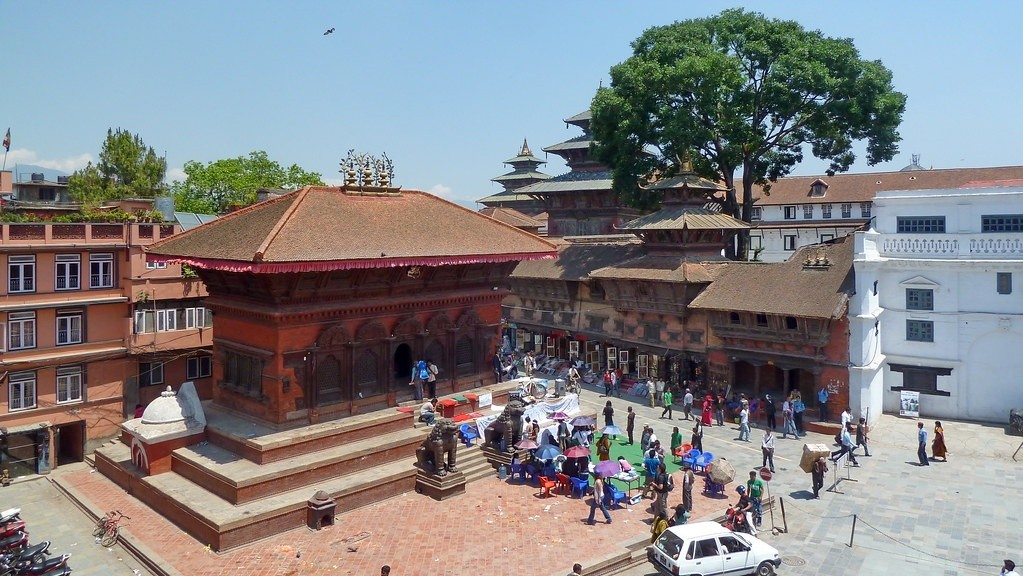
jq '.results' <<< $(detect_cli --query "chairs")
[704,477,723,498]
[460,424,477,448]
[674,443,713,477]
[508,464,627,510]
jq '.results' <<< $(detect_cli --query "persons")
[492,345,581,392]
[641,376,872,560]
[602,368,625,397]
[133,404,144,419]
[380,565,390,576]
[917,422,929,466]
[420,398,438,426]
[929,421,948,462]
[1000,560,1020,576]
[410,360,438,401]
[567,563,581,576]
[509,401,635,524]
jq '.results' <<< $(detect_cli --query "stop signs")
[759,467,771,481]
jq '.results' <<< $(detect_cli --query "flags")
[3,129,10,151]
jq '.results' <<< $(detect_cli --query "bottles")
[499,464,506,478]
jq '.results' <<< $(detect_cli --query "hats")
[643,423,648,427]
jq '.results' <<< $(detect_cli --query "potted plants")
[66,210,162,223]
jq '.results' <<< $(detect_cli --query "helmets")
[736,485,746,494]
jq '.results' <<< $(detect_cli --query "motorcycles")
[0,507,73,576]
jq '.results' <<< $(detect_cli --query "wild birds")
[323,28,335,36]
[297,550,301,558]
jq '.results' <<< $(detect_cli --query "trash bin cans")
[555,379,566,396]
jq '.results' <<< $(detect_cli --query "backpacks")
[660,472,674,491]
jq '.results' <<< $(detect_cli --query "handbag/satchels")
[600,439,609,455]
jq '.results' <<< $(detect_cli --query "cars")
[644,520,781,576]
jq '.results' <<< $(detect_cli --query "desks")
[589,465,640,504]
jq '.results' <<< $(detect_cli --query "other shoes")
[584,522,592,525]
[854,463,860,467]
[941,459,947,462]
[605,520,612,524]
[747,440,752,442]
[865,454,872,456]
[919,462,928,466]
[816,496,820,500]
[795,436,802,440]
[929,457,935,460]
[812,487,814,490]
[739,438,745,441]
[784,436,789,439]
[770,470,775,473]
[831,452,834,459]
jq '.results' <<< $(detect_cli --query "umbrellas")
[547,412,570,420]
[564,445,591,458]
[706,459,735,484]
[534,443,562,459]
[569,415,594,426]
[514,438,540,449]
[600,425,624,435]
[594,460,620,478]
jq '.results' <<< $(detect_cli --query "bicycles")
[565,377,581,394]
[516,374,547,399]
[92,510,131,547]
